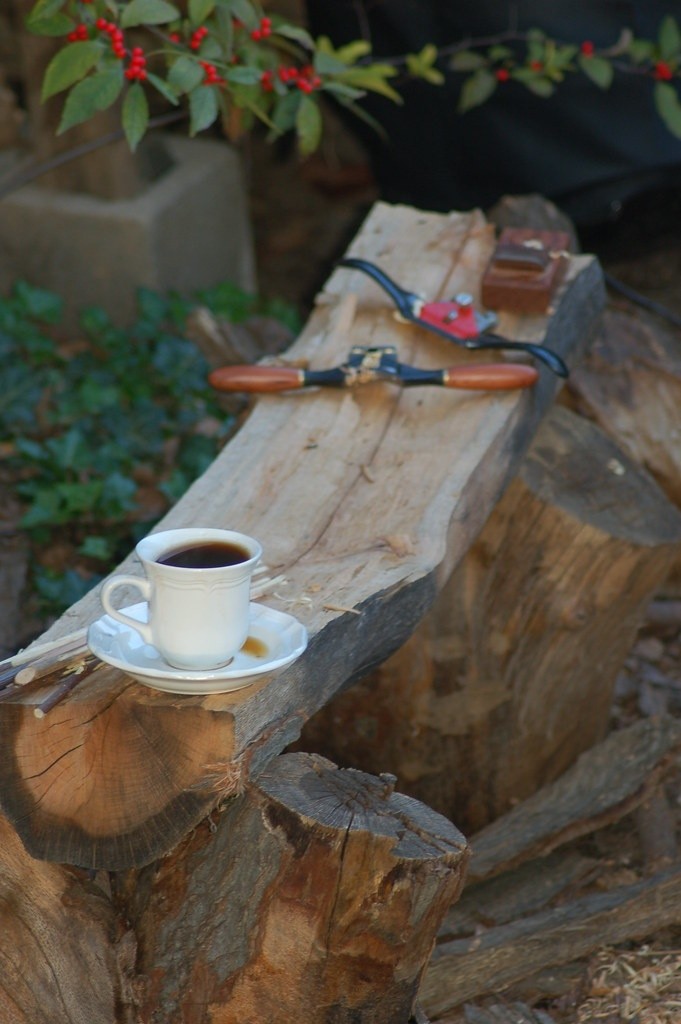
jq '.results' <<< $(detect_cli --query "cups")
[101,528,263,670]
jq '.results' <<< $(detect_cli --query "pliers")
[208,343,536,401]
[334,251,569,383]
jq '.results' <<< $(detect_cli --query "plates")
[86,600,308,695]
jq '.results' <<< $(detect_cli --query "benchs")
[0,202,680,1024]
